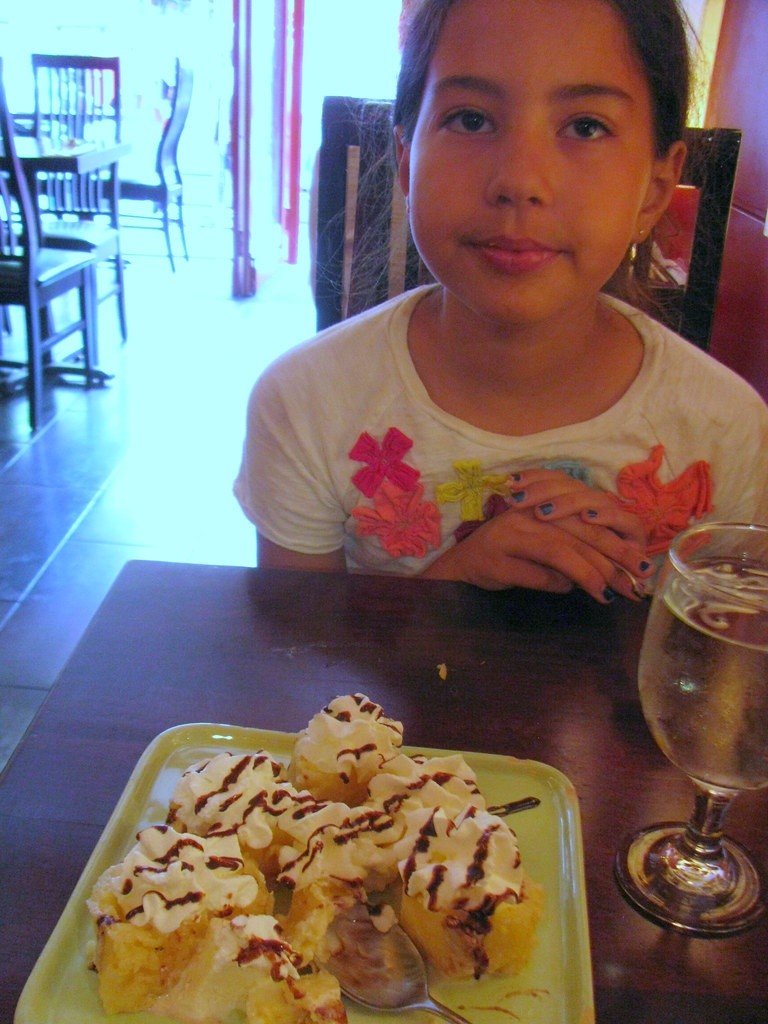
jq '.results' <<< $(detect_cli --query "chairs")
[315,95,742,354]
[0,53,192,434]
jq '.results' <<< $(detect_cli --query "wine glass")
[612,517,768,937]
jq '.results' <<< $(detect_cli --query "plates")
[12,717,599,1024]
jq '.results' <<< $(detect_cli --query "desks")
[0,134,127,399]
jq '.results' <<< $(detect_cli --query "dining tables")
[1,558,768,1024]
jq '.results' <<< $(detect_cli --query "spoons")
[312,902,473,1024]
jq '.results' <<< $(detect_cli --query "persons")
[238,1,767,605]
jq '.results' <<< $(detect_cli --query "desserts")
[86,691,547,1024]
